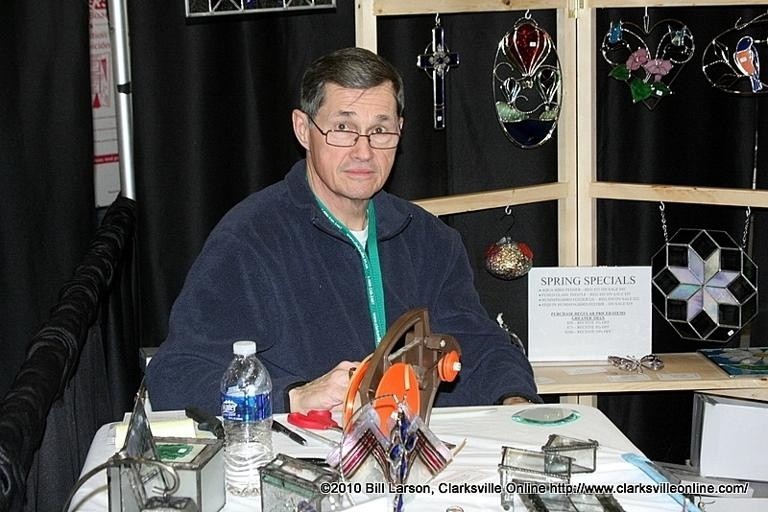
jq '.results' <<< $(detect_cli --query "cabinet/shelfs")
[531,347,767,511]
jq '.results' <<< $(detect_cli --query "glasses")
[301,110,401,149]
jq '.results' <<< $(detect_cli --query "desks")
[66,402,706,512]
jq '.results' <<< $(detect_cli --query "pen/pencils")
[270,420,307,446]
[286,422,339,448]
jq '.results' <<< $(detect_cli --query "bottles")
[215,339,271,500]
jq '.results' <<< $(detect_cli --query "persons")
[144,48,545,415]
